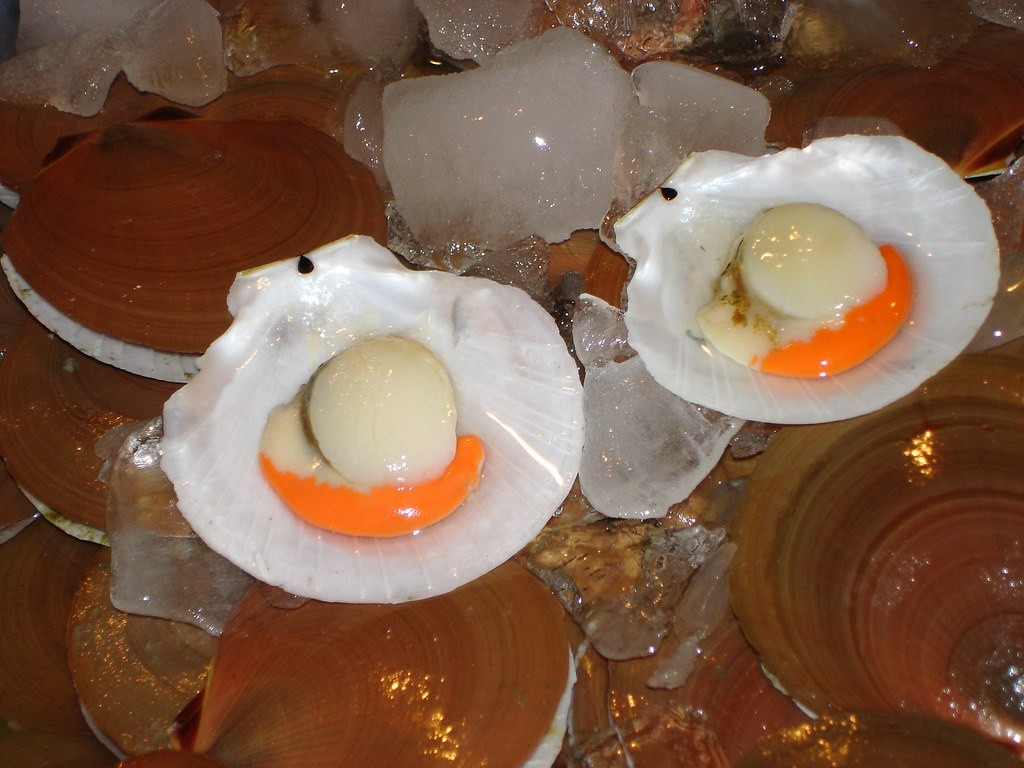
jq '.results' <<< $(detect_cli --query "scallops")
[3,22,1024,768]
